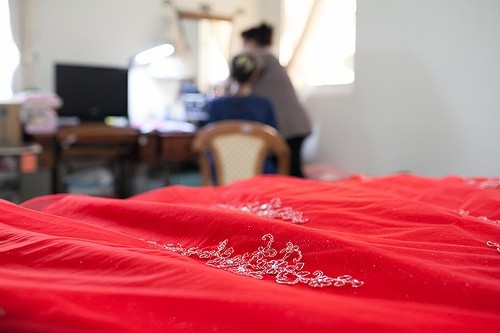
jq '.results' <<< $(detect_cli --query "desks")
[28,119,198,199]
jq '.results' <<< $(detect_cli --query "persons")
[204,53,279,188]
[219,22,313,179]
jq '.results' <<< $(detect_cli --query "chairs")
[192,119,294,188]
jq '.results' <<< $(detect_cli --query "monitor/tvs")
[55,62,129,118]
